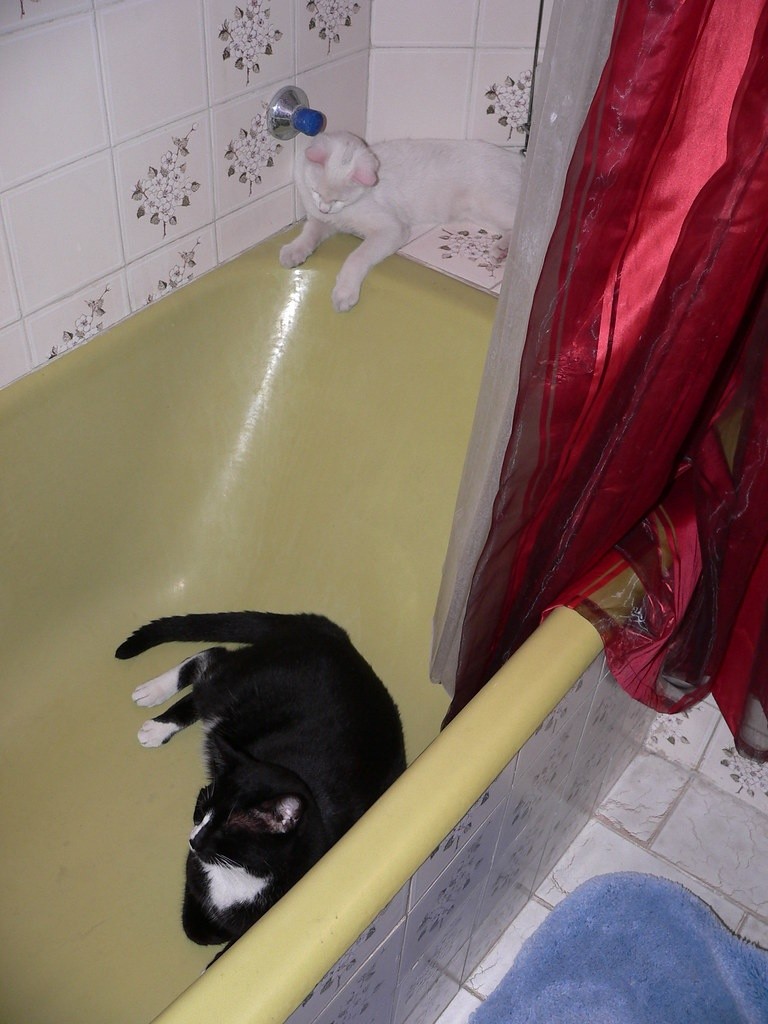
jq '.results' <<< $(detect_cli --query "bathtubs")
[0,218,671,1024]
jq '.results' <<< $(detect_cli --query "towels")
[464,867,768,1024]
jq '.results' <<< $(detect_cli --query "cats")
[115,609,408,976]
[280,129,527,313]
